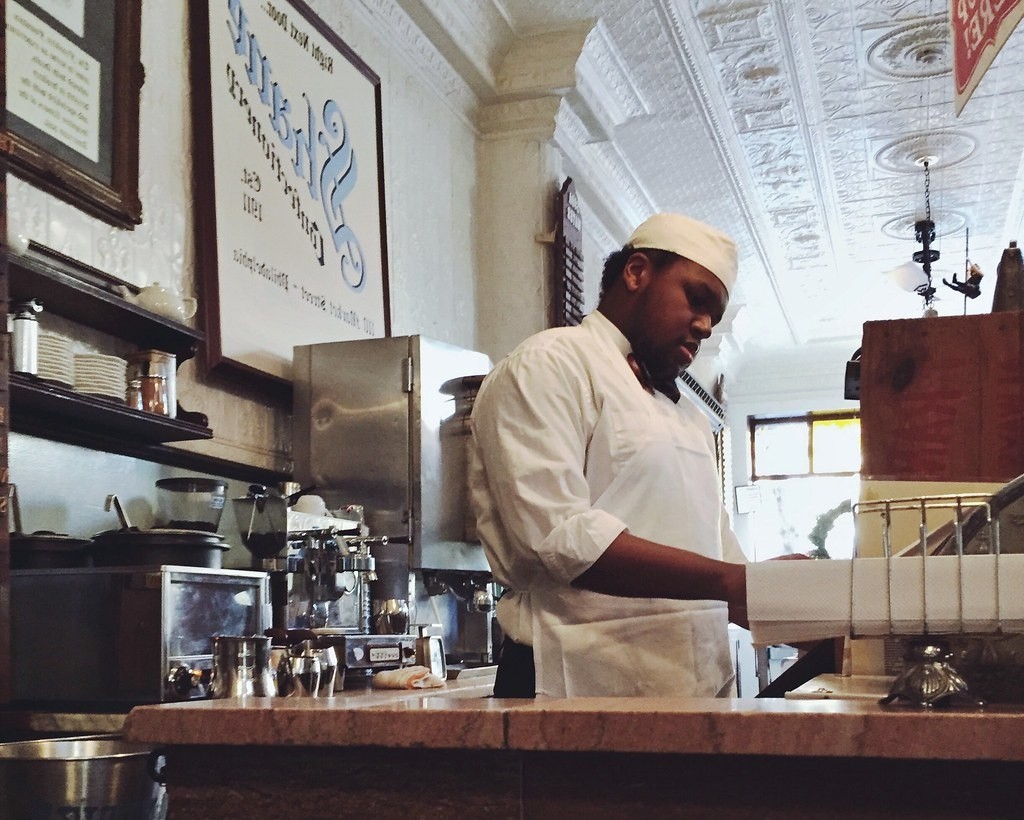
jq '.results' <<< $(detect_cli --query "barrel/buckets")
[0,733,169,820]
[211,633,276,703]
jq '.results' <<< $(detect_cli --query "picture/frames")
[0,0,145,231]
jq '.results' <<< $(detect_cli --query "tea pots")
[117,282,198,324]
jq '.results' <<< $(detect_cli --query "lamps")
[889,211,939,317]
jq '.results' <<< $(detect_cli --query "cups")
[8,230,29,256]
[169,654,214,701]
[272,636,345,699]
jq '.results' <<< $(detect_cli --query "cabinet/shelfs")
[3,243,209,465]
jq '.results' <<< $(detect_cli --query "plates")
[36,330,128,404]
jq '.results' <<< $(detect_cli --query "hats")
[620,212,738,299]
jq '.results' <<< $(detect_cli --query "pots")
[85,526,232,569]
[12,530,80,569]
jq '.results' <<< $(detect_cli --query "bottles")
[373,598,409,635]
[10,298,43,376]
[128,348,178,419]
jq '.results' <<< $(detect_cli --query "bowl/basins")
[156,478,230,532]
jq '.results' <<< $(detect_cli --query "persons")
[467,214,813,697]
